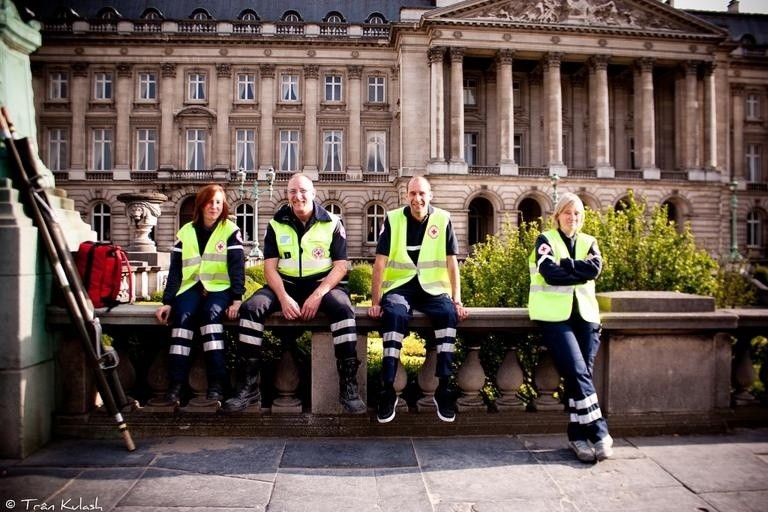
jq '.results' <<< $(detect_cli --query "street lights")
[237,165,277,259]
[724,174,741,268]
[549,171,561,212]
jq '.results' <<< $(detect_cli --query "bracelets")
[454,301,464,307]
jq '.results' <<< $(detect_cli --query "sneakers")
[164,382,187,403]
[594,432,614,460]
[375,389,399,424]
[568,438,597,462]
[207,383,223,402]
[432,383,457,423]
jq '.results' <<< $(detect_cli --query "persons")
[153,183,245,407]
[367,176,471,423]
[525,190,615,463]
[221,173,368,414]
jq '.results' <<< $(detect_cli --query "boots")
[335,357,369,416]
[224,357,264,413]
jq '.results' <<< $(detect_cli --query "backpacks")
[76,239,134,309]
